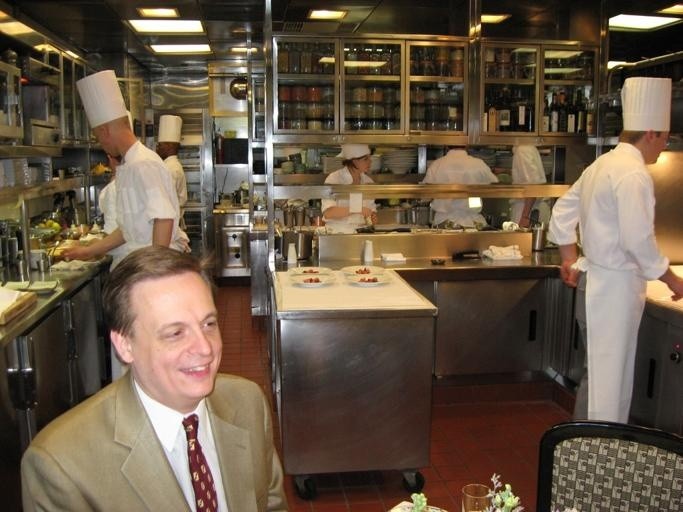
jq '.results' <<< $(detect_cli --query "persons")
[21,245,288,511]
[419,145,498,229]
[62,69,191,383]
[510,145,553,231]
[547,77,683,423]
[97,154,122,275]
[321,145,378,236]
[157,115,191,252]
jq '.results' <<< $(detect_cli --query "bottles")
[483,87,533,132]
[345,43,399,74]
[277,42,334,74]
[540,81,595,134]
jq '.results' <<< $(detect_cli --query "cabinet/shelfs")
[0,1,127,205]
[545,278,683,437]
[272,32,600,144]
[0,298,72,512]
[64,265,111,405]
[434,278,556,375]
[213,211,284,276]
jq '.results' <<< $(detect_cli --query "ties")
[182,414,218,511]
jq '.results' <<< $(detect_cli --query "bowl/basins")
[52,260,87,281]
[275,154,303,176]
[20,249,48,269]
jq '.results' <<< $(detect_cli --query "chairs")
[536,421,683,512]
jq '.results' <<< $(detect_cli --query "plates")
[5,280,57,292]
[342,265,382,275]
[291,274,334,288]
[290,267,334,277]
[349,273,386,287]
[384,147,418,175]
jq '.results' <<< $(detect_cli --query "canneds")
[540,46,595,77]
[410,46,464,77]
[345,80,401,130]
[409,81,462,132]
[278,82,333,130]
[484,47,535,78]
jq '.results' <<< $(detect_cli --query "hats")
[620,77,672,132]
[158,115,182,143]
[127,111,135,132]
[76,70,129,130]
[335,144,370,160]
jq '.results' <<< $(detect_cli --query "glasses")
[658,136,672,147]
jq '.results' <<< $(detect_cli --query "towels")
[51,256,107,270]
[483,245,523,261]
[503,221,519,232]
[381,253,406,262]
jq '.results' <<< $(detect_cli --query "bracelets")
[522,214,528,220]
